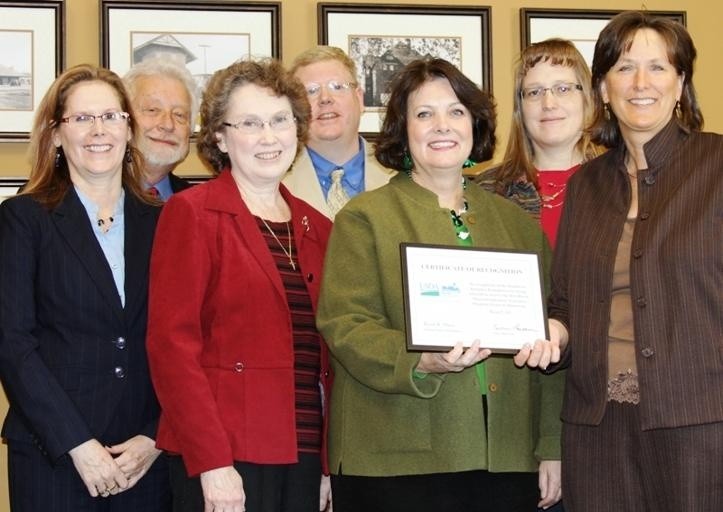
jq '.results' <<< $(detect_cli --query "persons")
[510,9,723,511]
[281,44,400,223]
[146,54,336,512]
[0,63,172,512]
[468,37,610,252]
[314,55,565,511]
[120,53,200,204]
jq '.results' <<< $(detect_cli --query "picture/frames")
[400,241,551,355]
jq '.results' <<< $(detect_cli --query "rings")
[106,483,116,493]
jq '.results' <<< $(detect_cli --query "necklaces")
[542,201,565,209]
[538,187,566,201]
[237,183,296,271]
[536,171,566,188]
[93,211,114,234]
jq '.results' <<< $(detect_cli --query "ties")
[148,187,159,198]
[327,169,351,216]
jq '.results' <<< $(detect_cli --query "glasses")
[60,111,129,128]
[307,80,357,97]
[223,116,297,134]
[521,83,582,101]
